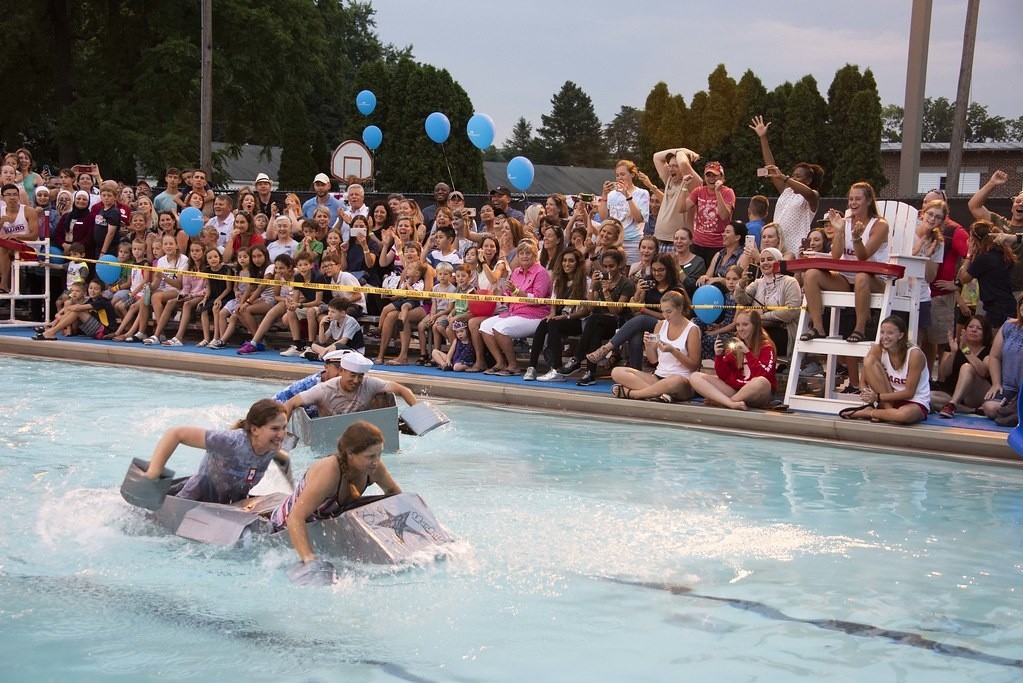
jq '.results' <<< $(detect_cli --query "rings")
[999,237,1001,239]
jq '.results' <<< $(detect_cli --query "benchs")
[115,314,609,358]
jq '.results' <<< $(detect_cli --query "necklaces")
[976,347,985,356]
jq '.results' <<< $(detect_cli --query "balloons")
[94,254,122,286]
[425,112,451,143]
[363,126,382,149]
[467,114,496,150]
[507,157,534,191]
[179,207,204,238]
[357,91,377,116]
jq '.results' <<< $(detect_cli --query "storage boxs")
[155,391,453,566]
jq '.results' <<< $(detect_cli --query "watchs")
[963,350,971,356]
[954,279,963,288]
[1016,233,1023,244]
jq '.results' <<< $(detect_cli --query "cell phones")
[718,338,737,348]
[56,201,67,210]
[582,194,593,202]
[801,238,810,255]
[466,208,476,216]
[79,166,92,172]
[757,168,768,176]
[177,294,189,300]
[648,334,660,343]
[275,202,287,210]
[608,182,620,190]
[44,165,50,177]
[745,235,755,250]
[747,263,758,282]
[642,280,656,289]
[597,272,610,281]
[351,228,367,236]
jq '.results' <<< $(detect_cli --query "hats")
[490,187,511,197]
[448,191,464,201]
[664,151,690,164]
[137,181,153,192]
[817,210,845,222]
[341,352,373,373]
[704,162,723,175]
[255,173,273,186]
[313,173,329,184]
[761,249,784,265]
[322,349,353,361]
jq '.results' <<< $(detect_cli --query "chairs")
[0,237,51,330]
[771,197,927,412]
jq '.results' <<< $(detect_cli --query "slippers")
[0,289,956,419]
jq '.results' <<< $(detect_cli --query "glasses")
[607,217,621,226]
[928,189,943,199]
[651,268,667,274]
[925,211,944,222]
[237,210,249,216]
[330,362,340,368]
[324,264,337,270]
[548,225,560,229]
[518,240,534,245]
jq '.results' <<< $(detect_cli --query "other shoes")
[994,397,1018,427]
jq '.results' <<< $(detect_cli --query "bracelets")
[589,288,593,293]
[397,251,403,256]
[96,178,103,182]
[590,257,597,261]
[877,393,881,402]
[681,188,688,192]
[299,303,303,309]
[851,238,862,242]
[246,301,251,305]
[784,175,789,182]
[962,347,969,353]
[602,199,607,202]
[101,250,106,254]
[966,254,973,260]
[514,288,519,295]
[363,249,370,254]
[678,269,684,276]
[650,185,657,191]
[480,261,487,266]
[369,232,374,236]
[626,196,633,201]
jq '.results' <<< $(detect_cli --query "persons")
[0,114,1023,425]
[270,420,401,562]
[144,397,292,504]
